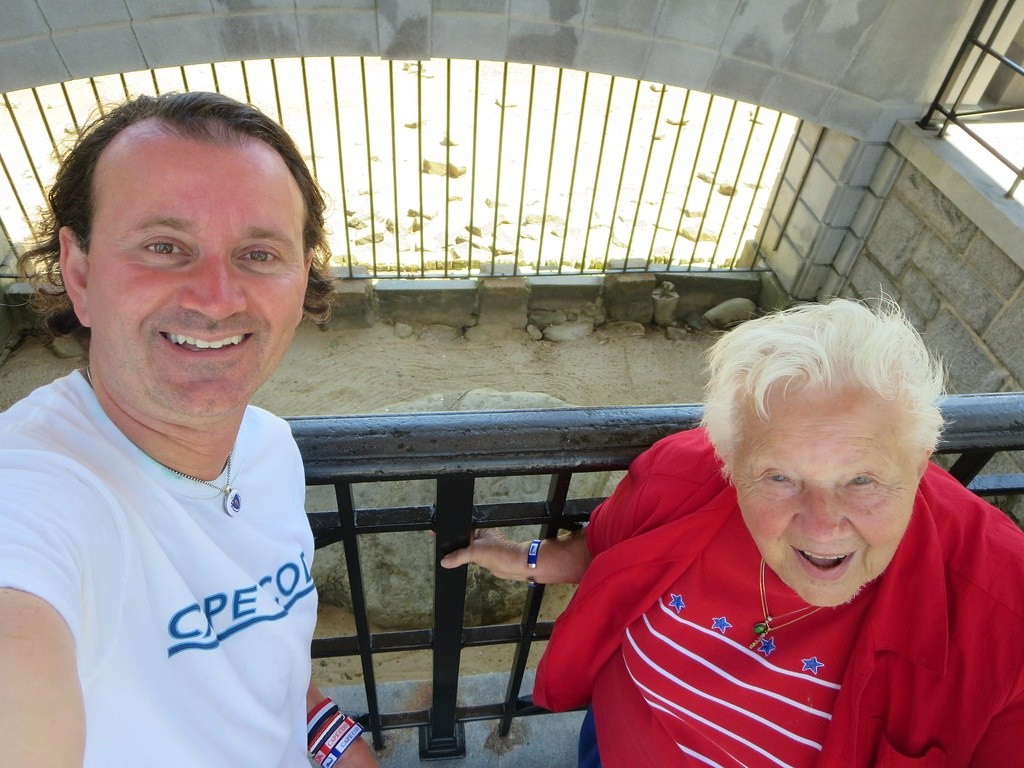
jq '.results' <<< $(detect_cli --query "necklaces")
[749,557,822,649]
[84,362,244,518]
[754,559,818,633]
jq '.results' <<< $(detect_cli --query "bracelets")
[313,716,355,765]
[526,539,541,588]
[321,721,364,768]
[305,696,341,748]
[306,710,346,755]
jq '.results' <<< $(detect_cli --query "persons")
[439,296,1024,768]
[0,91,384,768]
